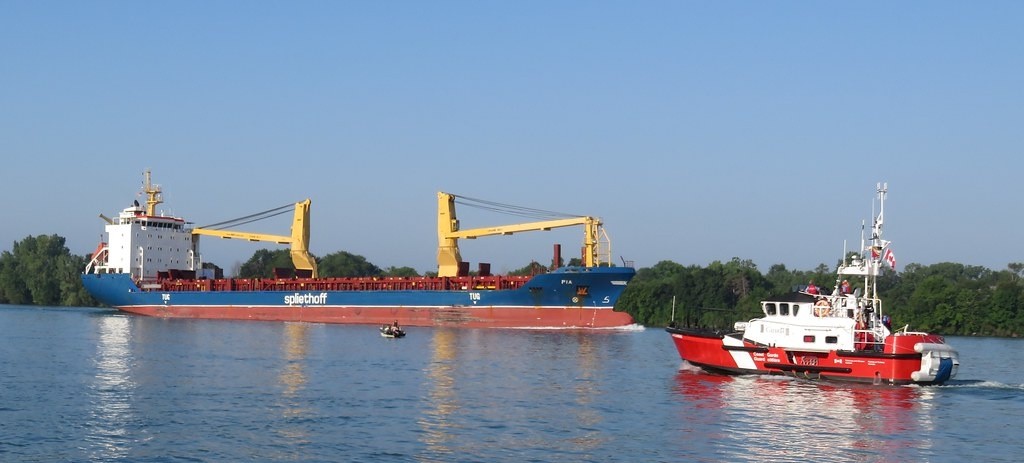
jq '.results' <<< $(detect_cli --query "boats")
[664,182,945,386]
[81,172,636,330]
[379,324,406,339]
[910,343,960,383]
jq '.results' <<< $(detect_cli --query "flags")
[882,248,896,270]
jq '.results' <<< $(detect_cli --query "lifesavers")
[815,300,830,317]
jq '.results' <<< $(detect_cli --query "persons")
[805,280,821,294]
